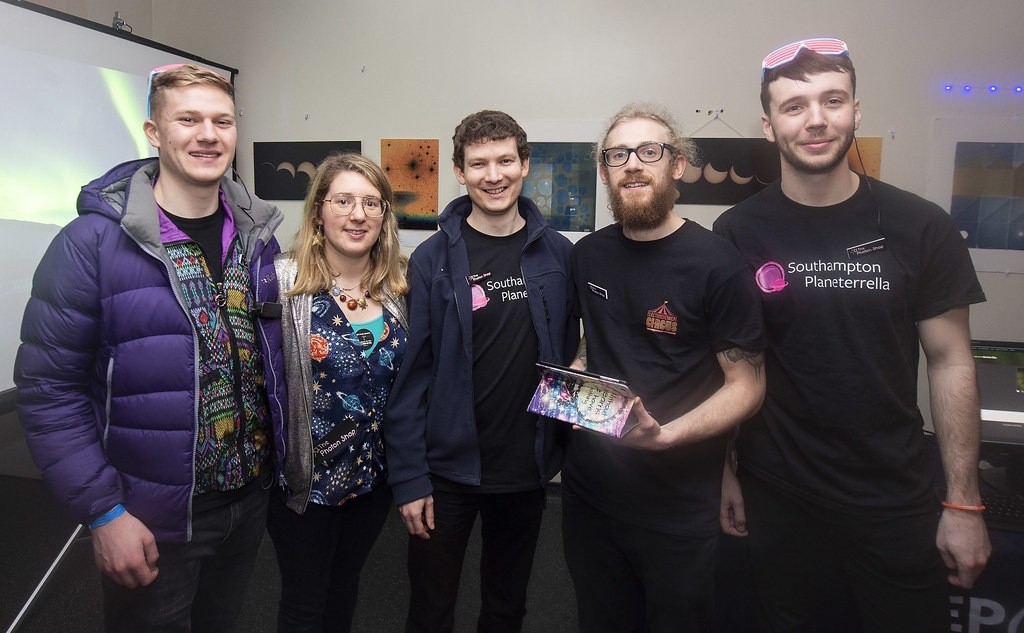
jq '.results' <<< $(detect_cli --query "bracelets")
[90,504,126,530]
[942,501,986,513]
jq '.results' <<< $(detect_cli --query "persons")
[713,38,990,633]
[386,111,580,633]
[274,154,409,632]
[14,66,285,632]
[561,105,767,633]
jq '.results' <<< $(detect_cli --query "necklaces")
[331,280,372,311]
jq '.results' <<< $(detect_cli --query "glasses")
[760,37,849,82]
[146,64,232,120]
[602,141,682,167]
[321,192,386,218]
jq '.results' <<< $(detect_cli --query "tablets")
[525,361,651,438]
